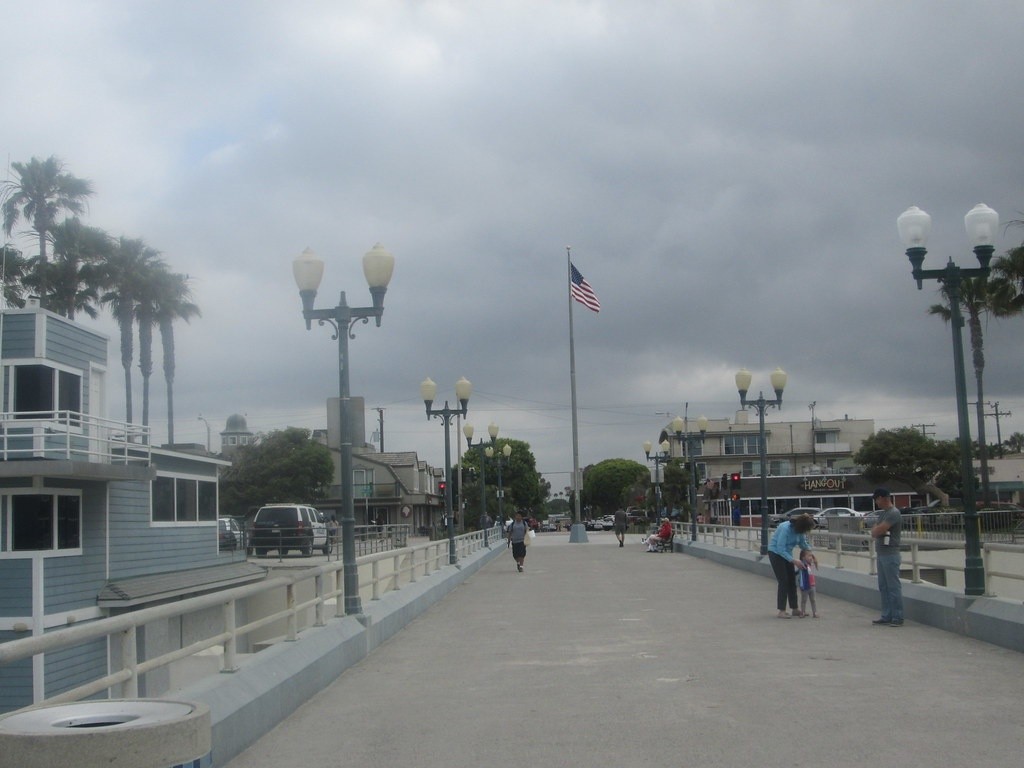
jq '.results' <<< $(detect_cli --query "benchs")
[656,532,675,553]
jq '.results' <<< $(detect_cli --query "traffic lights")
[438,481,446,489]
[731,472,741,489]
[721,473,727,489]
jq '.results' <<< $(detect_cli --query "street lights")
[463,420,513,546]
[421,375,472,564]
[643,439,669,532]
[897,201,1000,594]
[672,413,708,541]
[656,401,691,521]
[292,240,396,612]
[735,365,787,554]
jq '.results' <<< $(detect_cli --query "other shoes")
[871,618,892,625]
[890,619,904,627]
[517,562,521,570]
[519,566,524,572]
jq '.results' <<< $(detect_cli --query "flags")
[570,262,600,313]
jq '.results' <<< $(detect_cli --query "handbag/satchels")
[523,528,536,546]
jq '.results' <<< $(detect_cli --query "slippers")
[792,611,809,616]
[777,612,791,619]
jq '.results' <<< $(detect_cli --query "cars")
[559,523,572,530]
[523,517,558,533]
[812,507,864,529]
[862,499,1024,527]
[581,506,653,531]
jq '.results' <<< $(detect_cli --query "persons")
[641,518,672,552]
[507,511,529,572]
[697,513,705,523]
[494,516,514,530]
[481,512,493,529]
[614,506,629,547]
[768,513,816,618]
[734,507,741,526]
[795,543,820,618]
[376,511,384,532]
[328,515,339,543]
[869,488,904,626]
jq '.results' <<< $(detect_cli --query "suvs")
[217,517,245,549]
[772,507,822,525]
[247,503,334,557]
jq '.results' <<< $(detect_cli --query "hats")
[871,487,891,498]
